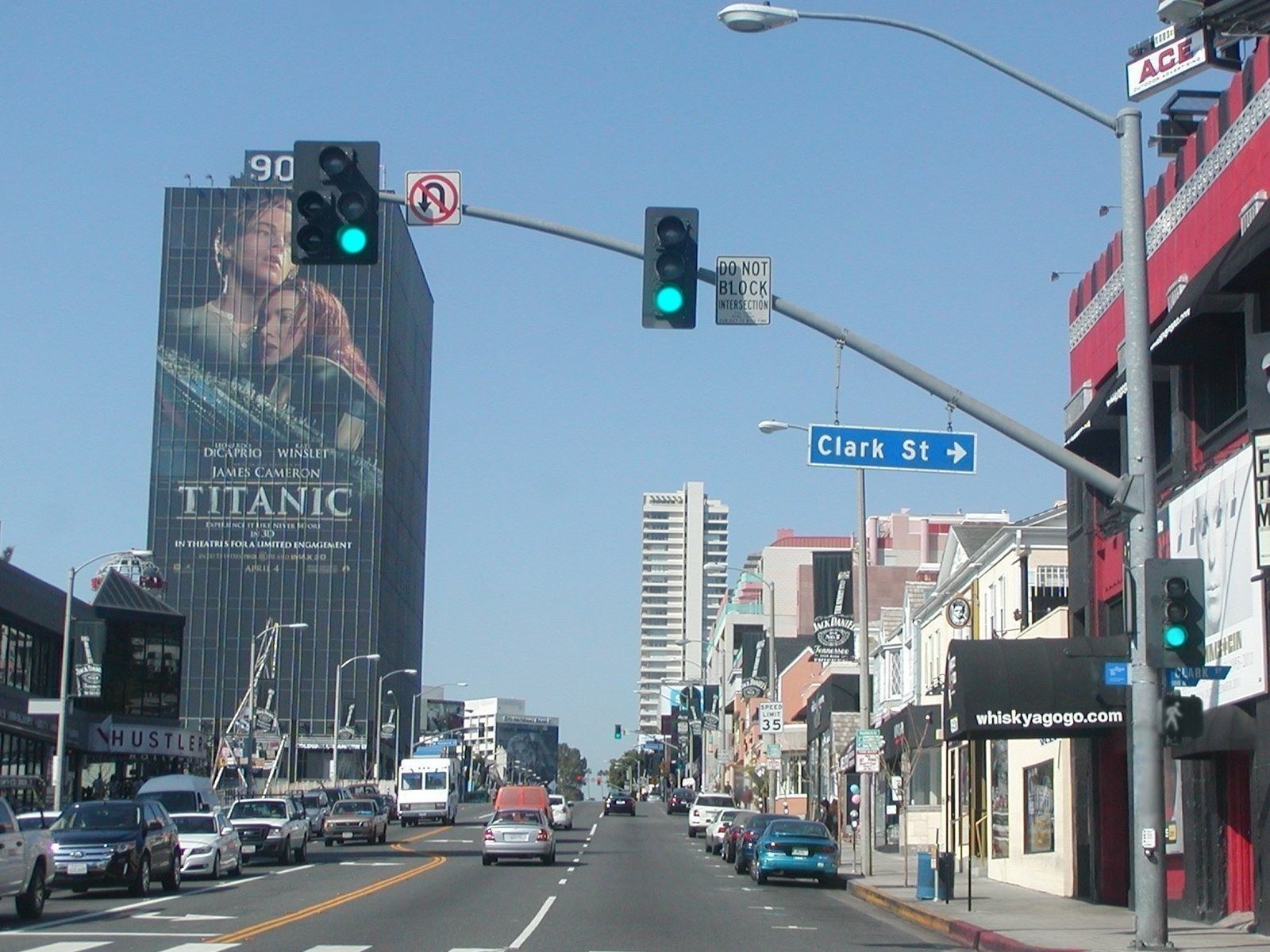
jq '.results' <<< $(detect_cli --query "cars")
[735,813,801,874]
[547,794,574,830]
[289,784,394,844]
[603,791,636,816]
[721,813,759,863]
[667,788,697,814]
[750,819,840,886]
[167,811,243,880]
[705,810,761,855]
[482,807,556,866]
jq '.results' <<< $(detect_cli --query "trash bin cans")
[916,852,957,900]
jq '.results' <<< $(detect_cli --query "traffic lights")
[641,207,699,328]
[291,142,380,264]
[1143,559,1207,669]
[615,724,621,739]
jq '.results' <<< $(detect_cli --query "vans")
[495,786,553,826]
[135,774,220,814]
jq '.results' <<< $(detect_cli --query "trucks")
[399,758,459,827]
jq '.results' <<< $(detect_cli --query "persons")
[254,282,387,464]
[953,601,966,625]
[817,801,833,834]
[164,200,299,394]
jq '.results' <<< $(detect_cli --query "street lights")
[410,682,469,758]
[632,637,726,803]
[53,549,154,812]
[714,2,1173,951]
[376,669,418,786]
[332,654,381,789]
[758,419,875,881]
[704,561,776,813]
[247,623,308,795]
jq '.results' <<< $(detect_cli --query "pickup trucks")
[0,797,55,920]
[227,798,312,864]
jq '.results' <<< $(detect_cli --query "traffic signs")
[809,424,978,475]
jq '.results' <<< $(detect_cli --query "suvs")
[45,801,181,900]
[688,792,738,837]
